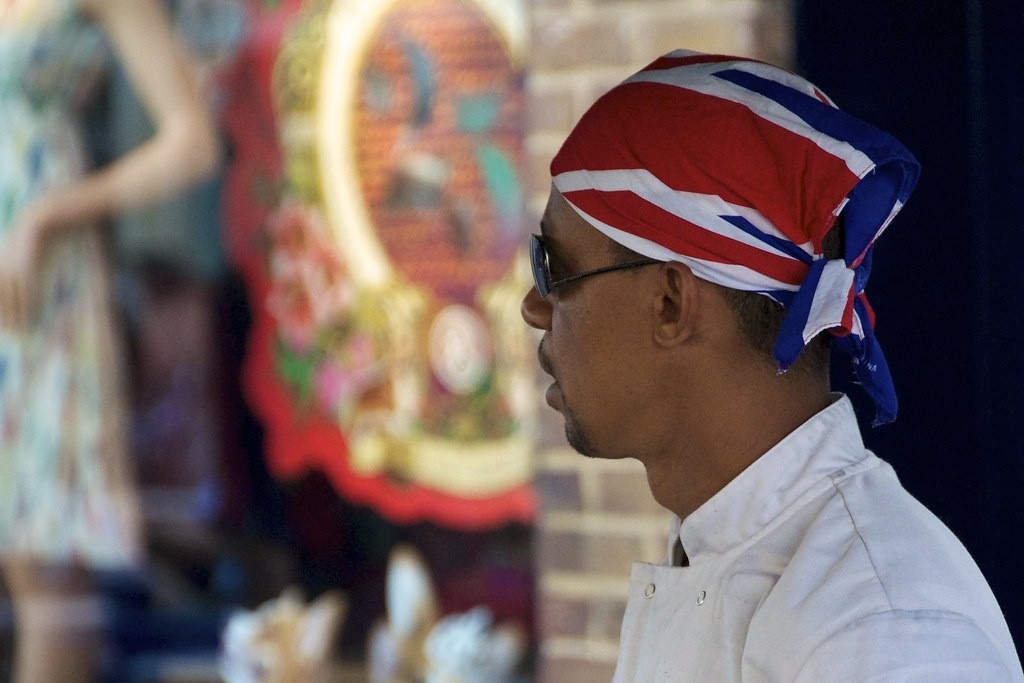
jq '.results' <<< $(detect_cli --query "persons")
[522,48,1024,682]
[0,0,233,683]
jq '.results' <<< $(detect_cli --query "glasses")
[529,230,667,299]
